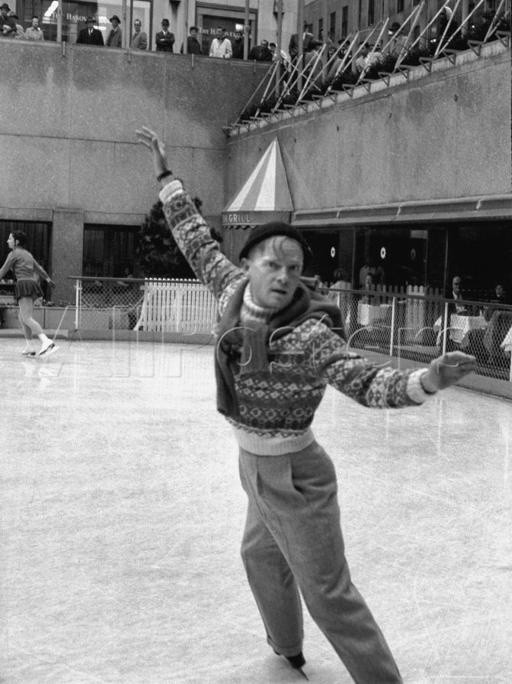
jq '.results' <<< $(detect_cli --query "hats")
[238,221,313,271]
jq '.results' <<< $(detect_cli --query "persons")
[430,5,461,45]
[132,118,482,684]
[322,264,352,314]
[1,228,58,355]
[25,14,45,42]
[75,15,287,64]
[443,271,471,318]
[1,2,16,35]
[359,255,386,285]
[358,271,376,294]
[482,280,511,321]
[384,20,412,57]
[288,26,386,95]
[8,10,24,39]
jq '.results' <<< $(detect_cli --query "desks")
[356,302,486,350]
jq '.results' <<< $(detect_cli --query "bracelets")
[157,170,171,182]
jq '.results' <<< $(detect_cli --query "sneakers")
[272,649,304,667]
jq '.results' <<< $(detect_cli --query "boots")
[39,333,54,354]
[21,339,35,355]
